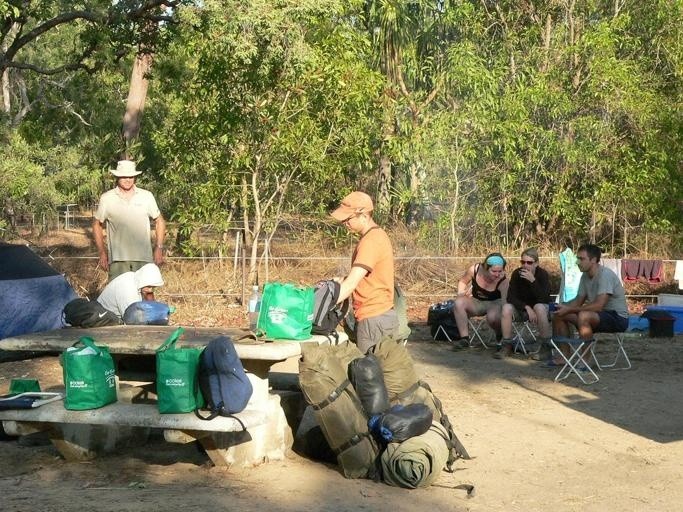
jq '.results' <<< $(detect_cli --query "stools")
[587,329,632,371]
[550,335,600,385]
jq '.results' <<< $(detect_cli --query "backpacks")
[295,342,381,479]
[309,279,341,336]
[365,336,458,462]
[427,299,469,340]
[123,302,171,326]
[393,286,410,339]
[197,336,253,414]
[62,299,122,327]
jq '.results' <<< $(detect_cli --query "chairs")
[467,317,495,350]
[513,278,553,356]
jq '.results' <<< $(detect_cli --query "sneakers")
[450,339,469,351]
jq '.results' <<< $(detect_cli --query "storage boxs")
[643,306,683,335]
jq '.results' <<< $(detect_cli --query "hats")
[329,191,374,221]
[109,160,142,178]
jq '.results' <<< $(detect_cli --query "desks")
[0,324,349,466]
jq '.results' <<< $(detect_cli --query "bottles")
[248,286,262,331]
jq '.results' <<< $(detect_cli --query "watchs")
[155,243,164,249]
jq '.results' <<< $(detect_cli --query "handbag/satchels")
[255,281,315,340]
[155,344,205,414]
[60,345,118,410]
[381,421,453,490]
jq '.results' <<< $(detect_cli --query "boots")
[530,338,554,360]
[493,339,514,359]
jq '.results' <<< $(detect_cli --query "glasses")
[520,259,536,265]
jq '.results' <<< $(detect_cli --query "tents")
[0,242,83,359]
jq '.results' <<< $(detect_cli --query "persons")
[330,191,400,355]
[493,248,552,359]
[91,159,167,305]
[548,243,629,371]
[452,253,509,348]
[95,262,165,324]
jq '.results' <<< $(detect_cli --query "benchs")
[0,385,264,444]
[0,391,262,467]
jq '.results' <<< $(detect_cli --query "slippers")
[248,328,275,342]
[567,364,594,373]
[228,332,264,345]
[544,359,573,367]
